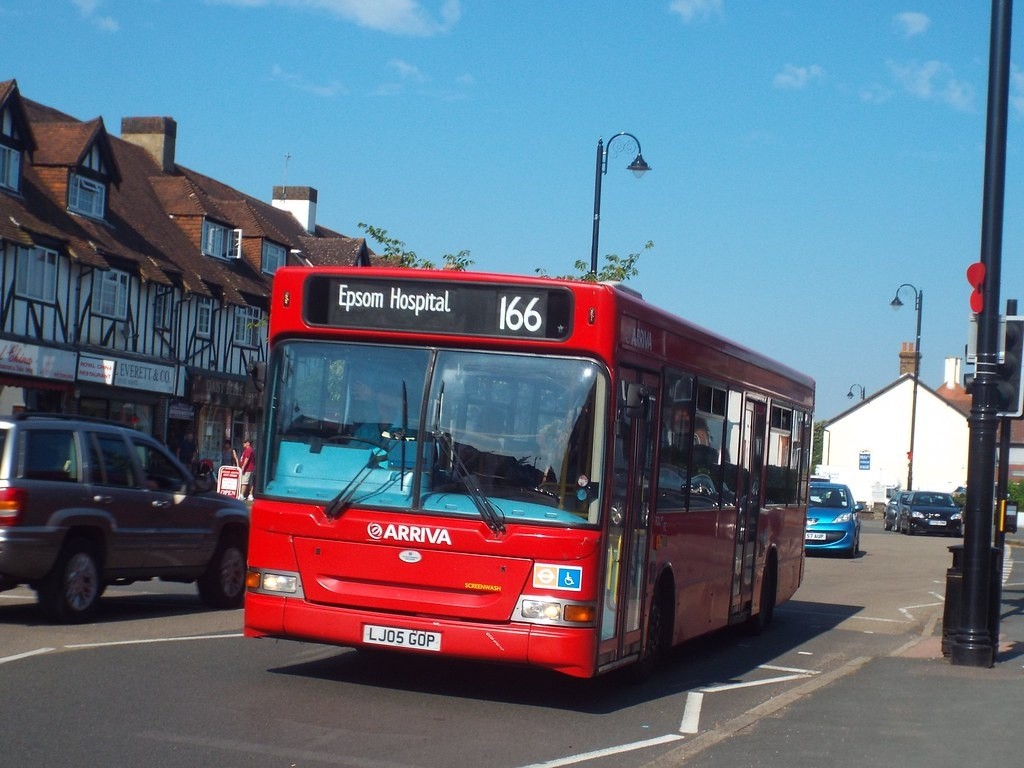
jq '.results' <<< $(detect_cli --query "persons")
[223,442,236,467]
[347,382,409,450]
[236,438,255,501]
[176,430,197,473]
[454,406,505,495]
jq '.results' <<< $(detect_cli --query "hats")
[243,438,252,445]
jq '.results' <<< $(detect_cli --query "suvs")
[0,409,250,626]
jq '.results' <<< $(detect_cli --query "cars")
[883,490,965,537]
[804,481,864,557]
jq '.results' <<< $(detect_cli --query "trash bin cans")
[941,543,1004,661]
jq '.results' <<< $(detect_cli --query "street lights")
[590,131,653,274]
[889,283,925,489]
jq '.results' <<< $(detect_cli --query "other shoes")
[238,495,244,501]
[247,495,254,501]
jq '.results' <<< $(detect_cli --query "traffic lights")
[992,315,1024,421]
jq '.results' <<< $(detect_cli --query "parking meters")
[999,500,1019,534]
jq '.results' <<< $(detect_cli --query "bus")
[240,263,820,685]
